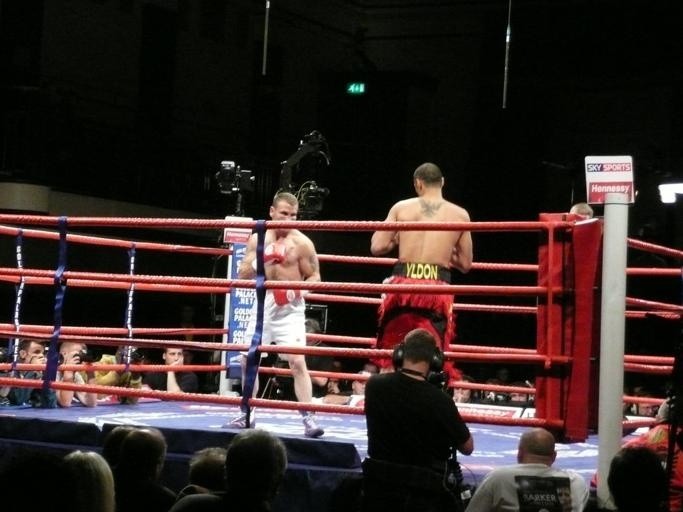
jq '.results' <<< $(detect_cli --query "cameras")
[56,353,64,365]
[74,349,102,364]
[130,350,145,361]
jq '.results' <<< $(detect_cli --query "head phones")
[392,340,447,373]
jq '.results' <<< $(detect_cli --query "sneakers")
[302,416,324,437]
[222,410,255,429]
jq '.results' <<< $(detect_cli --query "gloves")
[252,242,285,271]
[273,287,308,306]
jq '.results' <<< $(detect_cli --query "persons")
[224,193,327,438]
[203,317,394,411]
[452,373,536,403]
[172,304,208,341]
[369,162,475,423]
[607,384,682,510]
[3,425,288,511]
[463,427,590,510]
[1,339,201,410]
